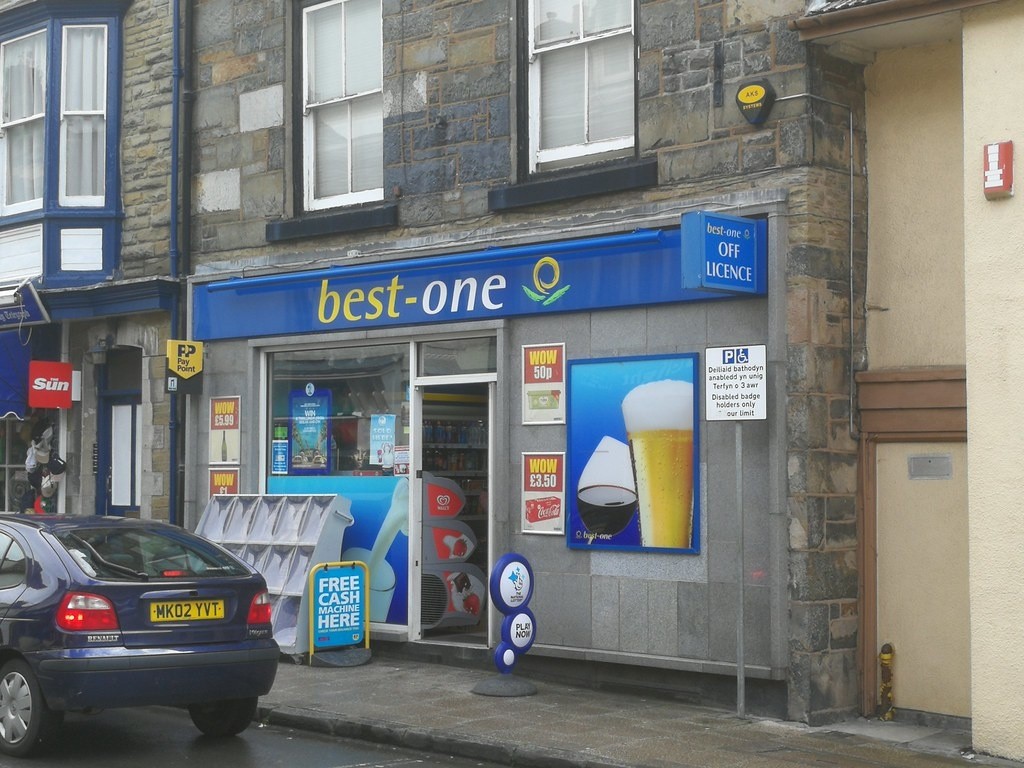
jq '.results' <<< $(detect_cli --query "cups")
[577,436,638,545]
[622,380,694,548]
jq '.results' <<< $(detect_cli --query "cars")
[1,502,281,758]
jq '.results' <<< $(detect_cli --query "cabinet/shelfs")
[421,393,488,570]
[195,492,355,665]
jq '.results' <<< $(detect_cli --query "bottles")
[221,431,228,461]
[423,418,488,516]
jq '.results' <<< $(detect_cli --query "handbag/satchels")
[18,416,67,516]
